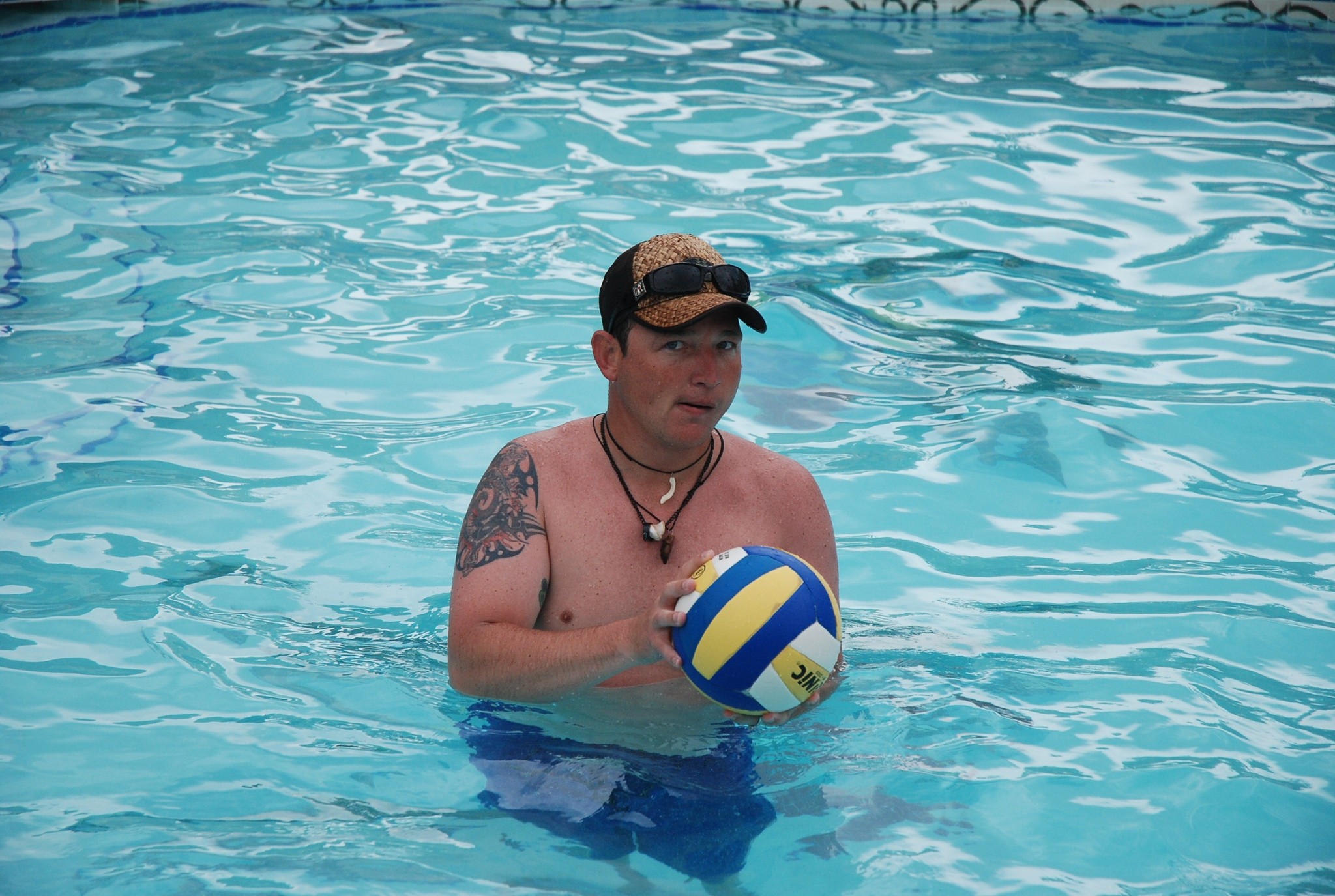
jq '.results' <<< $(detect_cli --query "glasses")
[609,262,751,334]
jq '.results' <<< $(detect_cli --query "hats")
[599,233,767,335]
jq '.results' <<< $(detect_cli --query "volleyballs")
[670,545,843,715]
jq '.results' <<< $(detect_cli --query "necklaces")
[589,413,723,563]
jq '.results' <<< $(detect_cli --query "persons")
[448,235,843,880]
